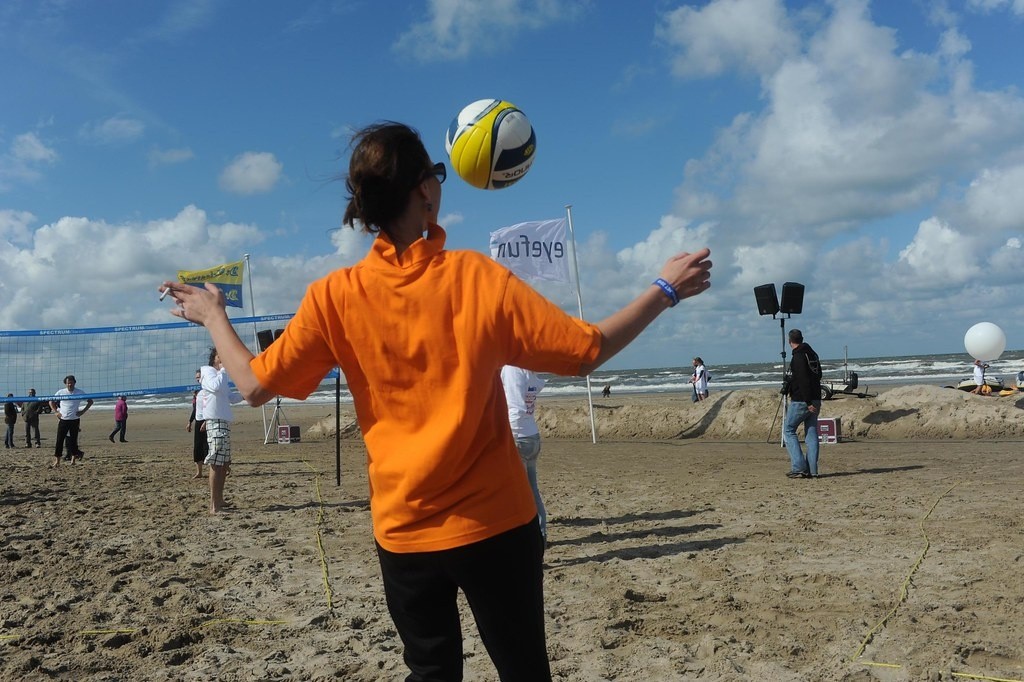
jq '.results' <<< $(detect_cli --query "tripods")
[263,395,289,444]
[767,314,790,448]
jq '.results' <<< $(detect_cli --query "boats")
[957,376,1004,392]
[1015,371,1024,390]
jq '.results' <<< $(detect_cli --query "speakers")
[257,330,273,352]
[274,329,284,340]
[754,283,780,315]
[781,282,805,314]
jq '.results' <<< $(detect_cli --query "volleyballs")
[445,98,540,190]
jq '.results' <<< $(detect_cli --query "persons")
[783,329,822,478]
[158,119,713,682]
[21,389,43,448]
[195,347,246,515]
[4,393,19,448]
[974,360,990,395]
[186,369,209,478]
[601,385,611,398]
[49,375,93,468]
[687,357,712,403]
[109,396,129,443]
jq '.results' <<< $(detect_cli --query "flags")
[176,260,244,308]
[489,218,570,284]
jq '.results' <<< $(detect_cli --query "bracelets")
[651,278,680,307]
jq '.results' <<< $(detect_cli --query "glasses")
[420,162,447,183]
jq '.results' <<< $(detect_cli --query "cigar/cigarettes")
[159,287,172,301]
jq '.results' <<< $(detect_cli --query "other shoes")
[786,471,798,477]
[120,439,129,443]
[64,456,72,460]
[36,444,40,448]
[109,437,115,443]
[6,445,19,449]
[79,452,84,461]
[25,445,31,448]
